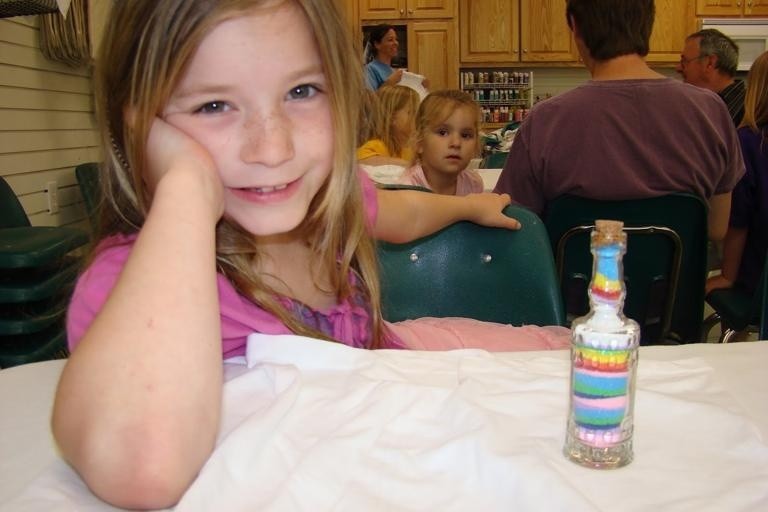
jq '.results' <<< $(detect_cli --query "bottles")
[464,71,528,84]
[478,106,530,123]
[562,219,641,468]
[463,89,527,102]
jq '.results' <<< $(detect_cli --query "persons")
[50,1,525,511]
[359,23,429,95]
[672,29,750,130]
[355,84,422,170]
[390,87,488,194]
[702,52,766,343]
[489,1,749,244]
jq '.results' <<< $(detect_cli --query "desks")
[2,342,767,510]
[354,165,505,194]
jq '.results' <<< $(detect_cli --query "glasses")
[681,53,709,65]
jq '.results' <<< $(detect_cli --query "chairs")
[74,159,115,239]
[706,238,766,342]
[0,176,89,364]
[478,150,510,169]
[542,190,710,347]
[368,205,564,326]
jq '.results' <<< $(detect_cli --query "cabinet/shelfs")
[458,70,536,132]
[350,0,460,20]
[344,20,458,131]
[693,2,767,25]
[458,0,577,69]
[580,0,703,68]
